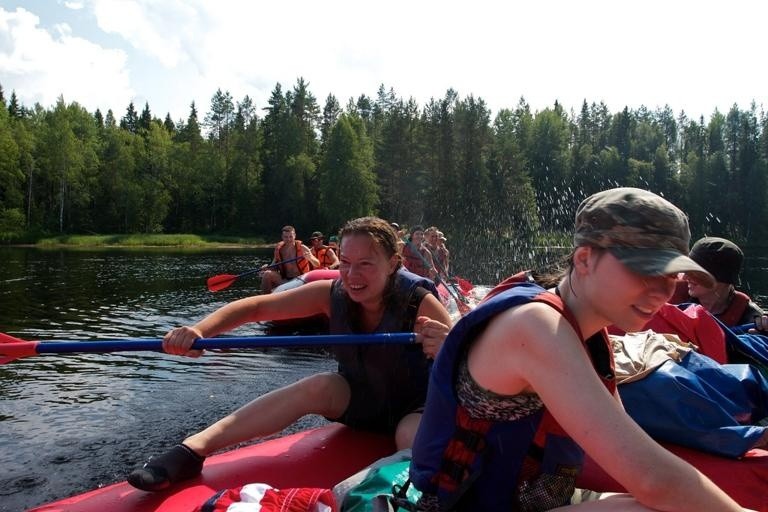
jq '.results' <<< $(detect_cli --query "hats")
[690,237,744,285]
[391,222,447,241]
[310,231,323,239]
[574,187,715,288]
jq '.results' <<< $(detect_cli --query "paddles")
[0,332,424,364]
[208,256,303,293]
[449,270,474,292]
[427,246,470,304]
[400,235,472,317]
